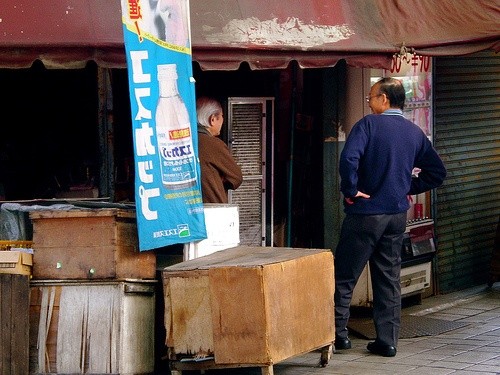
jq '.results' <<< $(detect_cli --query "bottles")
[155,64,197,189]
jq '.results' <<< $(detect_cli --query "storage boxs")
[30,207,158,280]
[0,250,33,274]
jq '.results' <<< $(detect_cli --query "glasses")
[366,95,389,102]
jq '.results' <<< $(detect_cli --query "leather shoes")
[335,337,351,349]
[367,342,396,357]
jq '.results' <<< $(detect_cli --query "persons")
[196,96,243,203]
[333,77,446,356]
